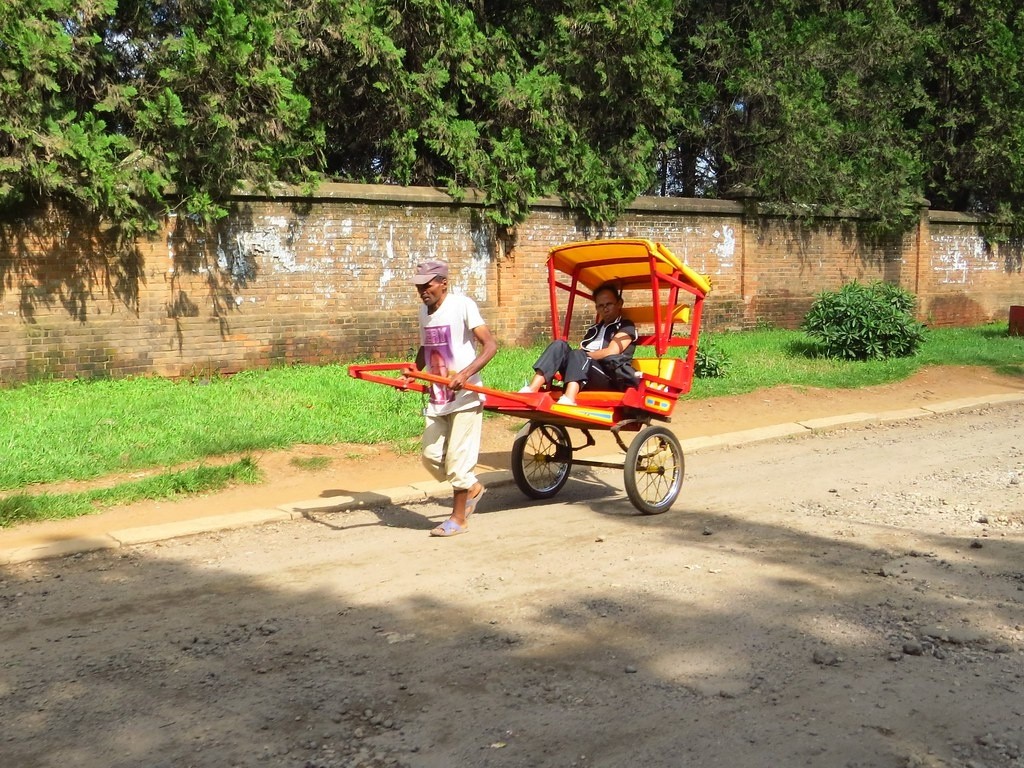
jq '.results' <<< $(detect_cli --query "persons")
[394,261,499,538]
[516,284,639,407]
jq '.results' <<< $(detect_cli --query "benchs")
[557,357,689,405]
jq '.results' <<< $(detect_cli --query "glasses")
[594,299,622,311]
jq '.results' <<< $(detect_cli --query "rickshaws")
[346,235,716,518]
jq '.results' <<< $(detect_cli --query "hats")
[411,259,449,286]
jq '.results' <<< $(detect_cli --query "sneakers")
[517,386,534,393]
[555,394,577,405]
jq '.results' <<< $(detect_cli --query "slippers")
[464,485,484,520]
[429,519,471,537]
